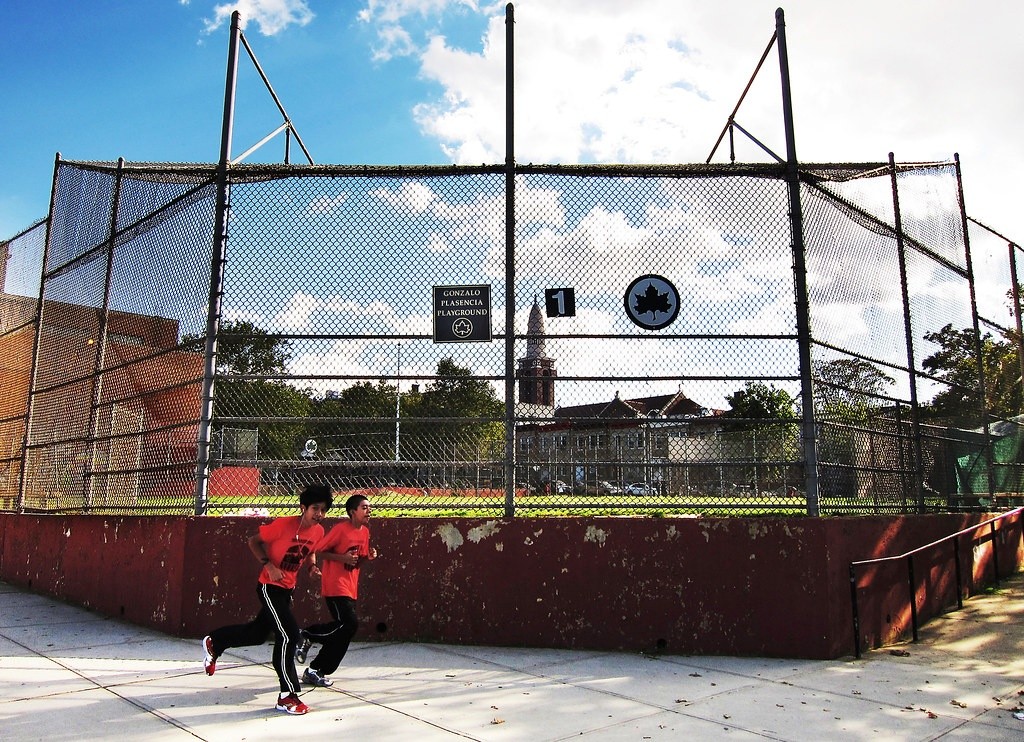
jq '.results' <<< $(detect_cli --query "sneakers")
[275,691,309,715]
[203,635,219,676]
[302,668,333,687]
[295,629,311,664]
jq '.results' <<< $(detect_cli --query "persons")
[295,494,378,688]
[202,481,334,714]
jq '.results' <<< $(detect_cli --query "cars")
[515,478,808,498]
